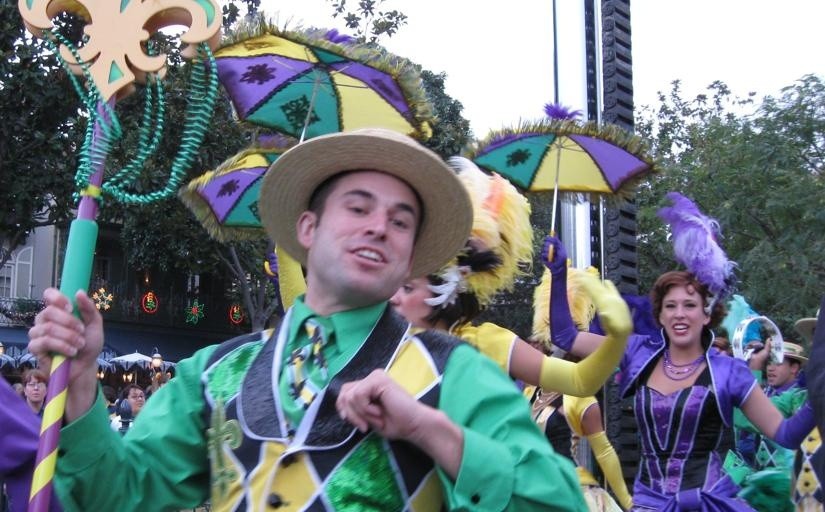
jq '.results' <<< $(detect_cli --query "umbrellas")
[470,119,654,250]
[173,143,288,242]
[201,24,434,146]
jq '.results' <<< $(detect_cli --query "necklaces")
[660,349,706,380]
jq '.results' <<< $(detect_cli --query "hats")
[258,127,473,287]
[783,341,810,364]
[793,307,822,342]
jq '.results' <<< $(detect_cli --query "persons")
[524,329,630,511]
[537,227,820,512]
[100,374,160,436]
[386,235,632,401]
[4,362,49,419]
[25,128,591,511]
[711,336,825,511]
